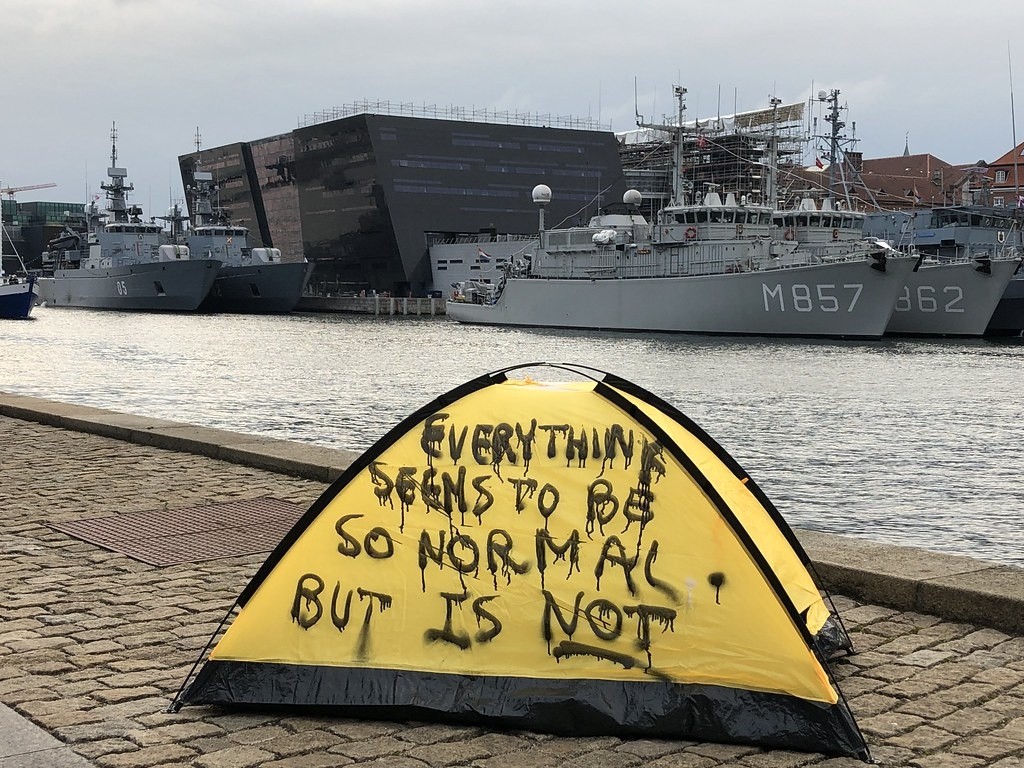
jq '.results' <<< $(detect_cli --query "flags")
[698,135,704,145]
[1018,196,1024,207]
[816,158,824,169]
[479,250,491,260]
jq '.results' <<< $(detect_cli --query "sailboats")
[0,181,40,319]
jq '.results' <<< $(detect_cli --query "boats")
[428,75,1024,343]
[21,121,223,314]
[185,126,316,316]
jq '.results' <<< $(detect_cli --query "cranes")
[1,183,57,200]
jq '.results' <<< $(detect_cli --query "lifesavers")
[685,227,697,238]
[784,230,796,241]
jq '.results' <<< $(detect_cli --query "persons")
[9,275,18,285]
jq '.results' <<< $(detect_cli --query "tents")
[162,362,874,765]
